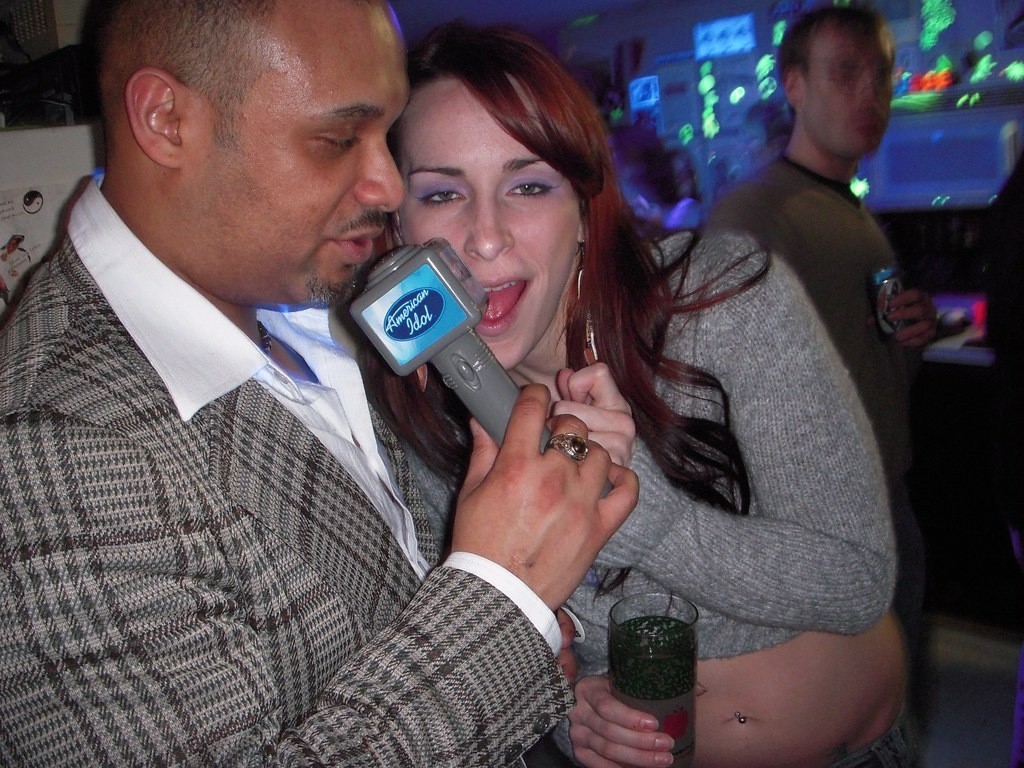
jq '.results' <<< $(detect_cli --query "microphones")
[348,237,554,454]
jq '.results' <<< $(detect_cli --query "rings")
[542,431,590,461]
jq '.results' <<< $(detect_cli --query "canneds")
[868,261,909,336]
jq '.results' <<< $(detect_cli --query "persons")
[1,1,642,768]
[697,0,939,741]
[388,18,927,768]
[972,143,1023,766]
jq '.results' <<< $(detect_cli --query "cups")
[609,593,699,768]
